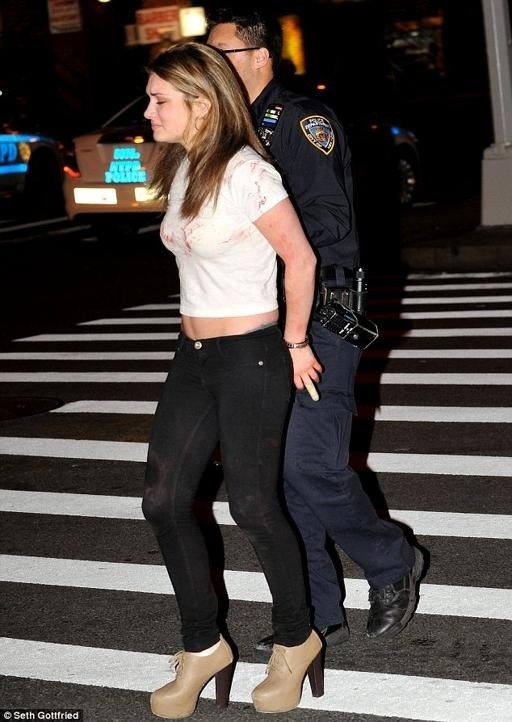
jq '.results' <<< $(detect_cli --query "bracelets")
[283,336,310,349]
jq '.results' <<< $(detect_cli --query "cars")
[60,91,421,227]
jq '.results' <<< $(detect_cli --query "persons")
[140,38,328,720]
[198,0,430,654]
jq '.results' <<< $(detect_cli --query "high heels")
[253,630,325,714]
[149,631,238,721]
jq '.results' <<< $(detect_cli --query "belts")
[314,264,354,283]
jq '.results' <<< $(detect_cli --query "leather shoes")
[256,621,349,653]
[366,539,427,641]
